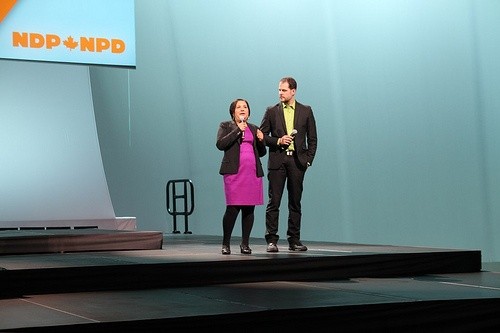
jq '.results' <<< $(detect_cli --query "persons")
[260,78,318,253]
[216,98,267,254]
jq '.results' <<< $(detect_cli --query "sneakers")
[289,242,307,251]
[267,243,278,252]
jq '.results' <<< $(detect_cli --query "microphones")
[240,116,245,139]
[282,129,297,150]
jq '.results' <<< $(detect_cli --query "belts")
[285,151,295,156]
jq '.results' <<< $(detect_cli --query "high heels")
[222,245,231,255]
[240,243,251,254]
